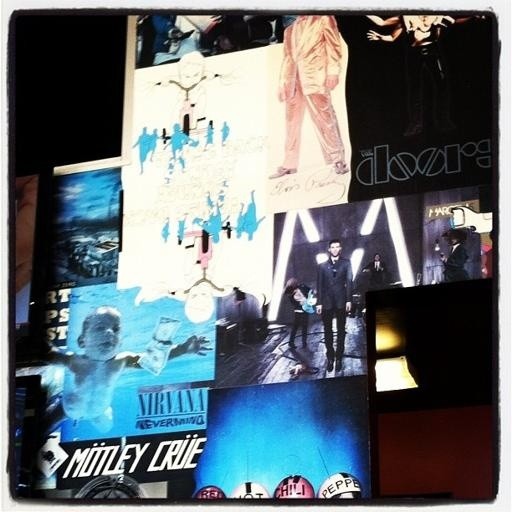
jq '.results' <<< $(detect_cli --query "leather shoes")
[328,359,334,372]
[289,342,307,348]
[335,161,348,174]
[268,168,296,179]
[336,359,342,372]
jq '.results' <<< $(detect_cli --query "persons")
[316,239,353,378]
[47,306,215,436]
[267,11,348,180]
[437,229,472,279]
[361,252,388,289]
[285,277,310,349]
